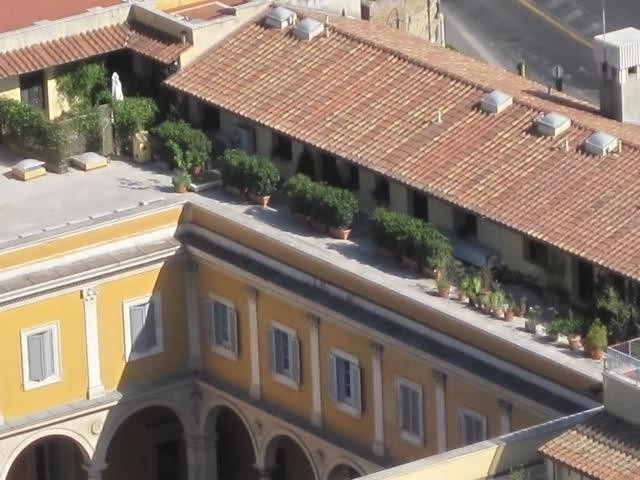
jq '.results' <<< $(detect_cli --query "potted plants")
[155,117,360,240]
[368,205,610,362]
